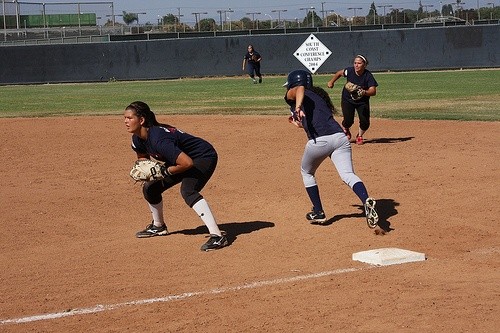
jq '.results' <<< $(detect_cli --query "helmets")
[283,69,313,90]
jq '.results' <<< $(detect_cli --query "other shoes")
[258,77,262,83]
[252,78,256,83]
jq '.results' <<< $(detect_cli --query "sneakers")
[305,206,327,222]
[344,129,352,140]
[200,230,233,251]
[355,134,363,145]
[135,219,169,238]
[364,197,379,229]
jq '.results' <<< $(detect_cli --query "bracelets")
[296,105,301,109]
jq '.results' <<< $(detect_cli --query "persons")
[328,54,379,145]
[282,71,379,229]
[123,100,229,252]
[242,45,263,84]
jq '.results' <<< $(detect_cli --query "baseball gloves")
[130,160,165,181]
[345,82,365,100]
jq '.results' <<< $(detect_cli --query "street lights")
[265,13,273,28]
[487,3,495,8]
[369,7,375,25]
[330,11,338,24]
[158,14,163,26]
